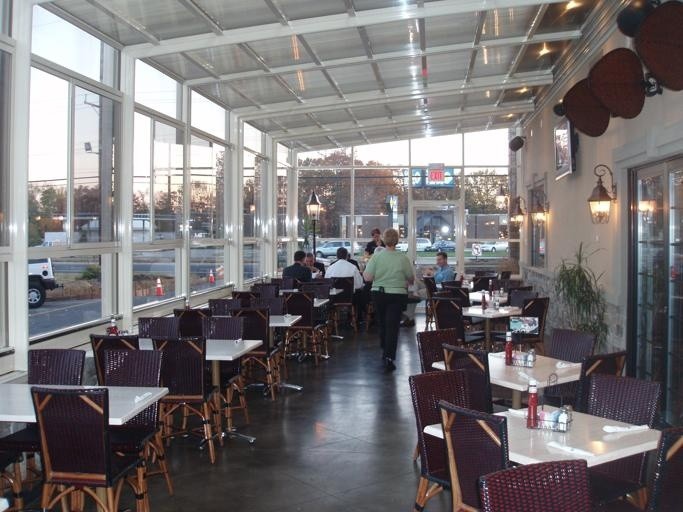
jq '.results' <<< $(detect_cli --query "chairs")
[408,268,682,512]
[0,263,374,511]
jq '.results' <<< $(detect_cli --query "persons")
[282,248,366,330]
[363,228,385,256]
[362,230,415,368]
[417,253,454,302]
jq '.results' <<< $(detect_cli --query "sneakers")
[383,357,397,372]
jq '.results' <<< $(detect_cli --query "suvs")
[312,240,359,258]
[276,239,313,262]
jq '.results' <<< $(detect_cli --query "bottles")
[208,269,215,283]
[479,279,505,311]
[185,300,190,311]
[558,404,572,430]
[525,378,538,428]
[155,278,163,295]
[108,317,119,337]
[505,330,513,366]
[527,348,537,368]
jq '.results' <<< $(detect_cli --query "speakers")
[509,136,524,151]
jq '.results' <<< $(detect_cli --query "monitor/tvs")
[554,115,580,181]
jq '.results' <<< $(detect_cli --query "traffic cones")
[209,268,216,284]
[155,277,165,296]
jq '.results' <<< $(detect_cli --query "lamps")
[510,195,526,231]
[586,164,618,225]
[530,189,549,224]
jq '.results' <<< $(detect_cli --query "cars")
[425,240,456,252]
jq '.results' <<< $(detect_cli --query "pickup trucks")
[28,257,57,308]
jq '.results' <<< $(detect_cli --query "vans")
[480,241,509,253]
[395,238,433,253]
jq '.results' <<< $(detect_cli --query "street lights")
[306,189,322,263]
